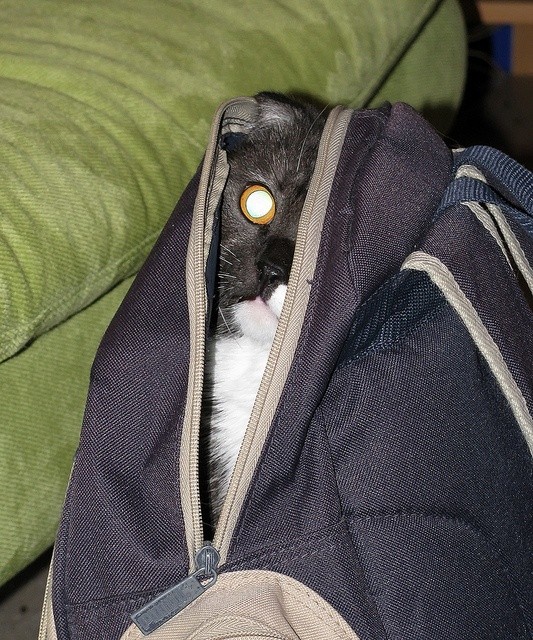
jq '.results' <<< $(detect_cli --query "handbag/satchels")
[38,98,533,639]
